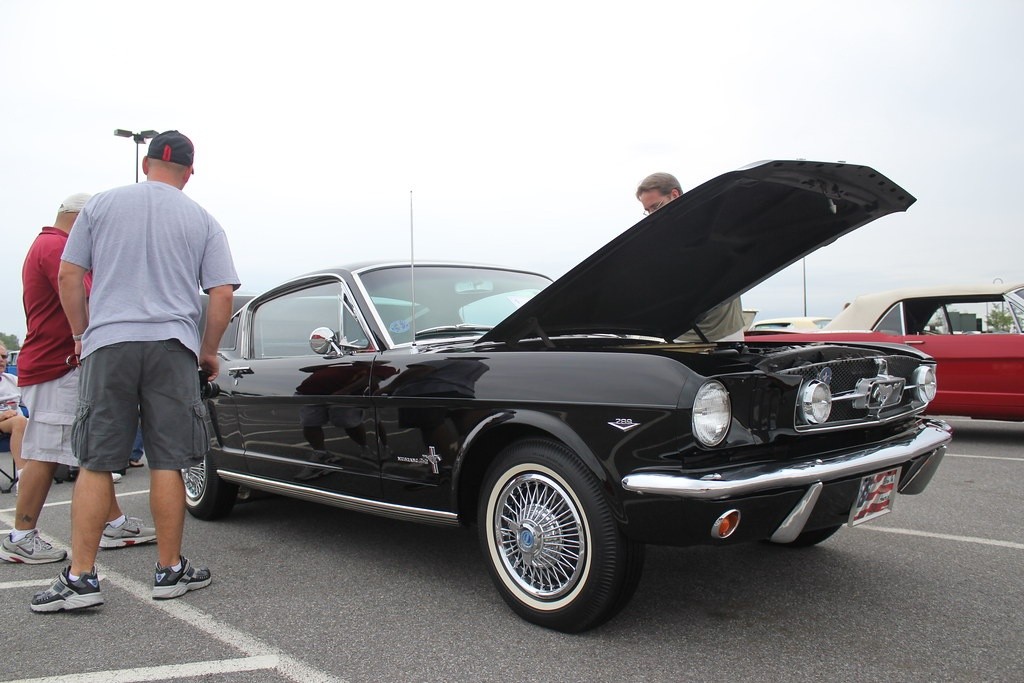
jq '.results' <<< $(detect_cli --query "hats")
[147,128,194,174]
[57,192,90,212]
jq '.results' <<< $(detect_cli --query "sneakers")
[111,471,121,483]
[0,528,66,563]
[150,556,211,598]
[30,564,104,612]
[97,514,157,548]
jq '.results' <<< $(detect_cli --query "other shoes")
[15,468,22,496]
[54,471,75,483]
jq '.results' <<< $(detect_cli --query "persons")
[30,131,241,612]
[0,192,157,565]
[1,339,29,496]
[294,356,490,488]
[635,172,745,341]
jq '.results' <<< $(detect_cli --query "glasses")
[643,193,666,215]
[0,353,7,359]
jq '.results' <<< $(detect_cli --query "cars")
[821,284,1024,427]
[746,315,832,335]
[179,156,953,632]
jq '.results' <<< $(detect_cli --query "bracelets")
[72,334,83,341]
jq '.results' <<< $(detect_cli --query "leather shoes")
[128,459,144,467]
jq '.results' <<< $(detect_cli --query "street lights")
[112,128,159,185]
